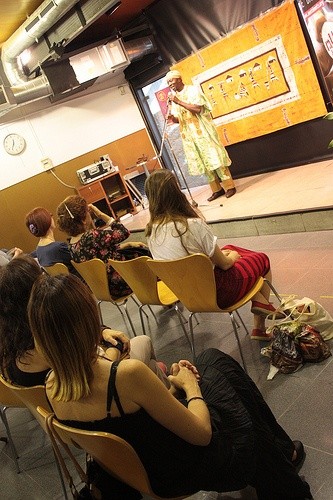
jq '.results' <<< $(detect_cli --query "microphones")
[167,87,177,102]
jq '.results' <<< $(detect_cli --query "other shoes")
[291,439,304,467]
[226,187,236,198]
[250,329,272,341]
[251,301,276,318]
[208,188,225,201]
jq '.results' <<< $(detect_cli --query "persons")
[57,195,154,300]
[27,271,313,500]
[0,255,169,387]
[24,207,90,291]
[164,70,236,201]
[144,168,281,341]
[0,247,22,267]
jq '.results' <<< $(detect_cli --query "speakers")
[122,58,160,84]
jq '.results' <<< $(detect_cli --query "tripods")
[158,101,223,209]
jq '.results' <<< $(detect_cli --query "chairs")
[0,253,293,500]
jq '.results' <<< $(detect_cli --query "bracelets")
[185,397,205,406]
[107,217,114,226]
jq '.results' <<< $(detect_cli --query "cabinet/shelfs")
[78,171,138,221]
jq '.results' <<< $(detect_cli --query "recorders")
[76,160,115,185]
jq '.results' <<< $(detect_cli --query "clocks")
[4,134,26,155]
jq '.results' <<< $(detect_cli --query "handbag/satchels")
[43,413,115,500]
[264,296,333,341]
[260,323,331,374]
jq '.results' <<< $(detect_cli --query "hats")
[165,70,181,82]
